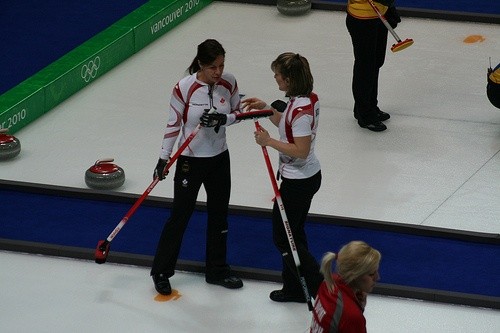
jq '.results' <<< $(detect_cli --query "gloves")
[154,158,170,181]
[200,109,226,133]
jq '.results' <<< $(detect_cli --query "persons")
[150,39,243,295]
[309,241,381,333]
[241,52,324,303]
[487,62,500,108]
[345,0,401,131]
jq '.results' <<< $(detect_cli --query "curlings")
[0,128,21,158]
[85,158,125,190]
[276,0,312,16]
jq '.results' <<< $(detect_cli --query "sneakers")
[353,107,390,132]
[151,273,171,295]
[204,274,241,289]
[270,288,308,303]
